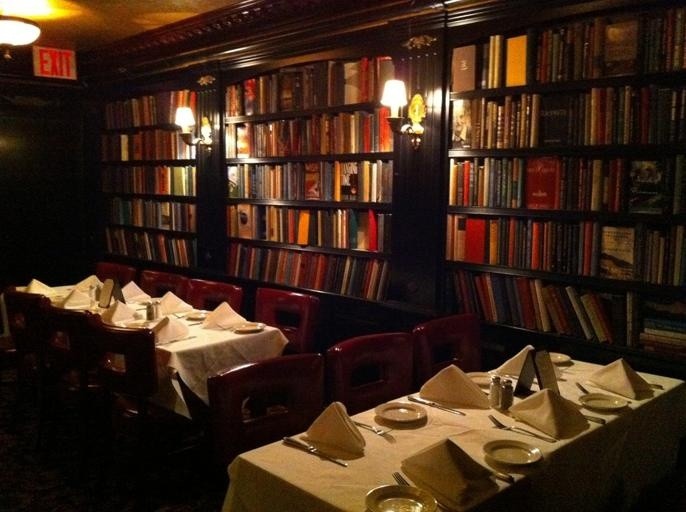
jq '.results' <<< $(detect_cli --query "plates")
[375,402,427,422]
[126,296,267,334]
[548,352,572,363]
[482,440,543,466]
[464,372,496,387]
[364,484,438,511]
[578,392,628,413]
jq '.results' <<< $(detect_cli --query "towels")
[510,386,584,439]
[589,359,653,400]
[401,438,496,506]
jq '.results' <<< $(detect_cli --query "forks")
[577,383,589,394]
[486,415,558,443]
[348,419,393,436]
[392,471,448,509]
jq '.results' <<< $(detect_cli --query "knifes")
[282,437,349,468]
[407,395,466,416]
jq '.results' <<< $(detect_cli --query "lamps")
[0,14,43,61]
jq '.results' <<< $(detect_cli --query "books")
[95,89,198,267]
[442,8,686,358]
[221,57,397,299]
